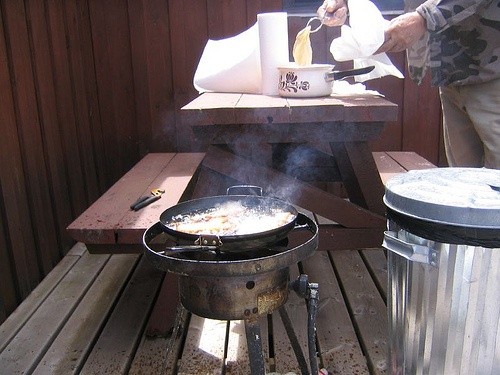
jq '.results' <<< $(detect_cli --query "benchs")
[66,153,206,254]
[372,150,438,188]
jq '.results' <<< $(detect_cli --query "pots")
[277,63,375,97]
[159,185,299,256]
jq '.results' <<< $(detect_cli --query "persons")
[317,0,499,169]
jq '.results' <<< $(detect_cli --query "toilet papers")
[193,11,290,96]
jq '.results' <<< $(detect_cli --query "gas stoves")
[142,211,319,321]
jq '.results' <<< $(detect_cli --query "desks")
[0,197,392,375]
[144,83,401,341]
